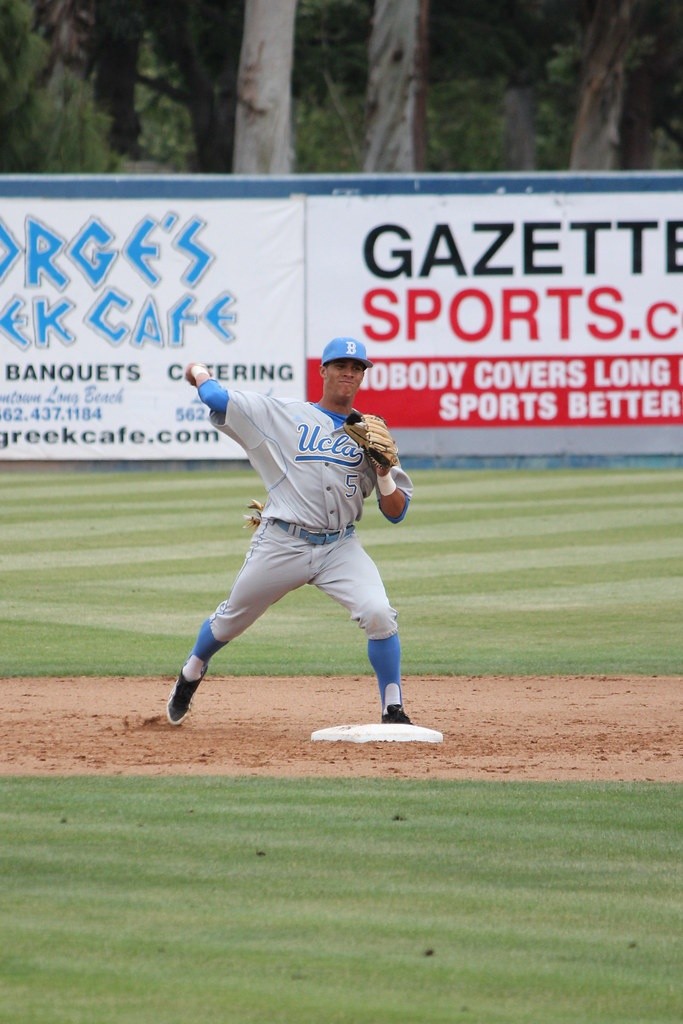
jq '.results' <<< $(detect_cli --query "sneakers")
[167,665,208,725]
[381,704,413,725]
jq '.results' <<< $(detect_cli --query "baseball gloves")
[343,411,399,469]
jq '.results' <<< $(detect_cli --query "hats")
[321,337,374,368]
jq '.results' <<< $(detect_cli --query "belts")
[274,519,355,545]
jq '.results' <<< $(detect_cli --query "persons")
[166,338,412,726]
